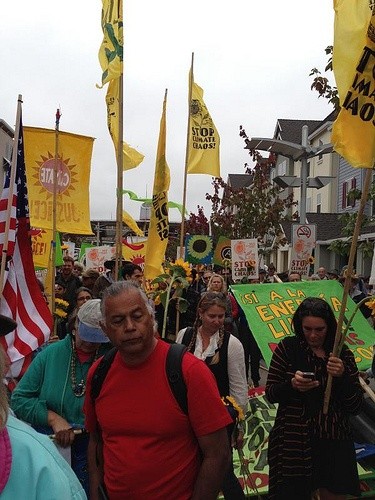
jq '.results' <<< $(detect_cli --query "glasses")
[199,292,227,308]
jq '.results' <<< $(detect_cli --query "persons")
[0,252,375,500]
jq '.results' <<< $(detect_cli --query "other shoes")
[253,380,259,386]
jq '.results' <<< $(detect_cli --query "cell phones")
[303,372,316,381]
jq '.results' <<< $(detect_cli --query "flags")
[1,0,375,392]
[186,65,221,178]
[16,125,96,236]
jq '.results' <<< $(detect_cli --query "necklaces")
[70,335,100,399]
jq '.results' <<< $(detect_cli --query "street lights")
[243,125,337,225]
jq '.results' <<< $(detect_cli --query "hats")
[77,298,110,343]
[83,269,100,279]
[104,255,131,269]
